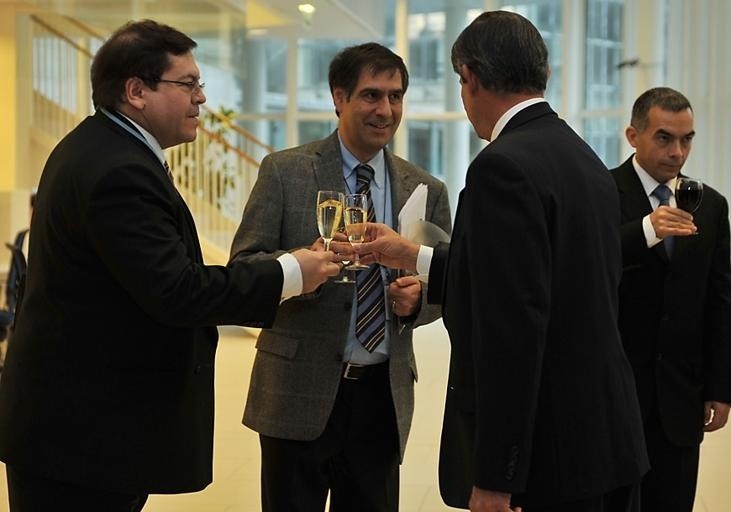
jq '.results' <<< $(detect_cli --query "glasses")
[155,77,207,90]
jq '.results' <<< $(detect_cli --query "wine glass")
[674,178,704,237]
[343,192,368,272]
[316,188,335,267]
[338,262,352,287]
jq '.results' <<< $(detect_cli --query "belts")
[336,361,391,382]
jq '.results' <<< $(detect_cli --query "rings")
[391,300,396,310]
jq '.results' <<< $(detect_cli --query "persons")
[1,19,342,511]
[607,87,731,510]
[227,44,452,512]
[343,12,654,512]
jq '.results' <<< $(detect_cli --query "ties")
[163,158,175,186]
[652,184,677,258]
[354,165,386,352]
